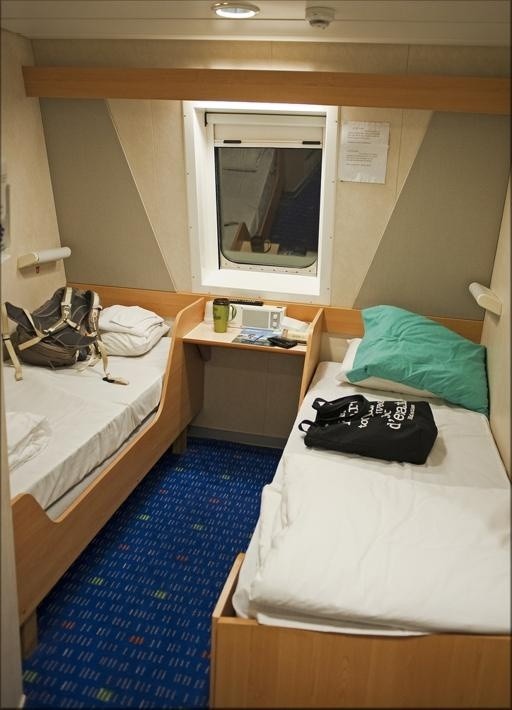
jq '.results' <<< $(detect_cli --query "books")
[232,326,280,347]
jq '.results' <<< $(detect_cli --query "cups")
[213,298,230,333]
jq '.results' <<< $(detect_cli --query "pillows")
[99,322,171,357]
[336,336,440,398]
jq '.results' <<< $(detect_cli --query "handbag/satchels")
[299,394,438,464]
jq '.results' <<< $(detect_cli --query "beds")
[210,305,509,709]
[2,279,204,665]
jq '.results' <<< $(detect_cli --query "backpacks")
[1,286,129,387]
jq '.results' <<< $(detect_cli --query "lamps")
[211,2,258,18]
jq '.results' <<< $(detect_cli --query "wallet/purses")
[268,336,297,348]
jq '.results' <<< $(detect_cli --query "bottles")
[251,235,271,253]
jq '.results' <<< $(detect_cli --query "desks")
[182,319,307,361]
